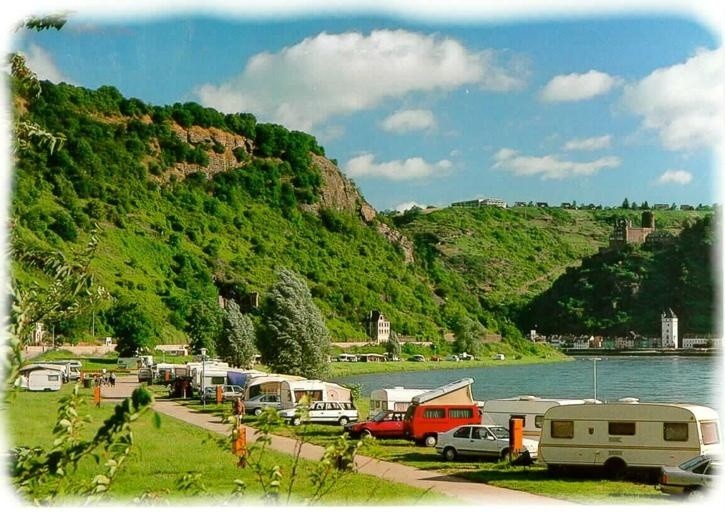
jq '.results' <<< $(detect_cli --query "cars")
[658,454,722,504]
[435,425,539,462]
[344,410,408,441]
[240,393,280,417]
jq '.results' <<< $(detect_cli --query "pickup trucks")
[138,368,152,383]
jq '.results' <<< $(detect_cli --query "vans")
[275,400,358,426]
[201,385,244,403]
[404,404,495,447]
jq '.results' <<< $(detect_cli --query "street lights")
[200,348,209,405]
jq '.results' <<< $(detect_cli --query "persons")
[102,367,116,387]
[233,397,245,427]
